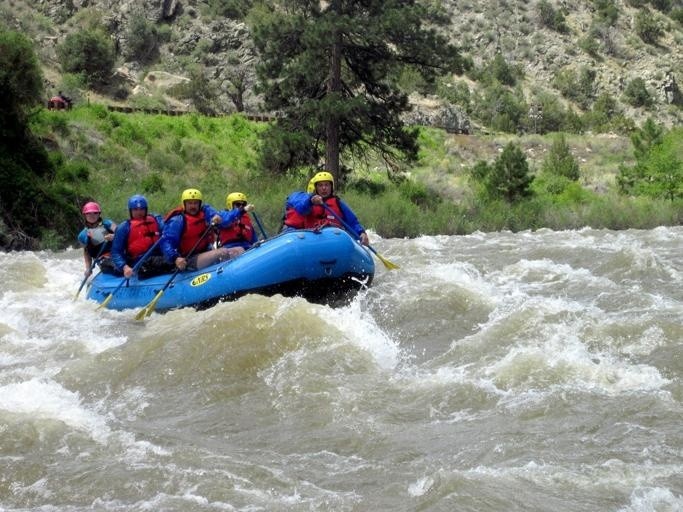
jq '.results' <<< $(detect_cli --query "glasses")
[233,202,246,208]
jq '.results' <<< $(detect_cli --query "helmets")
[182,189,202,211]
[307,178,316,193]
[227,193,248,211]
[128,195,148,217]
[314,172,334,192]
[82,202,101,214]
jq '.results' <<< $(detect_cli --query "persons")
[78,170,372,279]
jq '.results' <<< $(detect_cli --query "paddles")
[96,234,164,311]
[135,220,216,322]
[320,201,400,269]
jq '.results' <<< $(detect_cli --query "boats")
[75,228,378,316]
